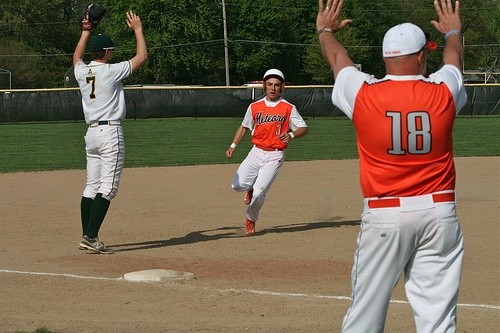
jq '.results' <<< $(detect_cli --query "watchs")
[317,27,334,34]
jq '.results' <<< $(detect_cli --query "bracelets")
[289,131,295,138]
[230,142,237,148]
[443,28,461,39]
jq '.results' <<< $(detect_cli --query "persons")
[316,0,469,332]
[73,9,148,253]
[226,68,309,234]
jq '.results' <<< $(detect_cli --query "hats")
[90,32,115,51]
[263,68,285,83]
[382,22,438,58]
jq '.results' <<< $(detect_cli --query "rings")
[325,5,331,9]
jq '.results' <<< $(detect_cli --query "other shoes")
[244,188,254,205]
[245,217,256,235]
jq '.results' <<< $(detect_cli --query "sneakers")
[79,235,113,255]
